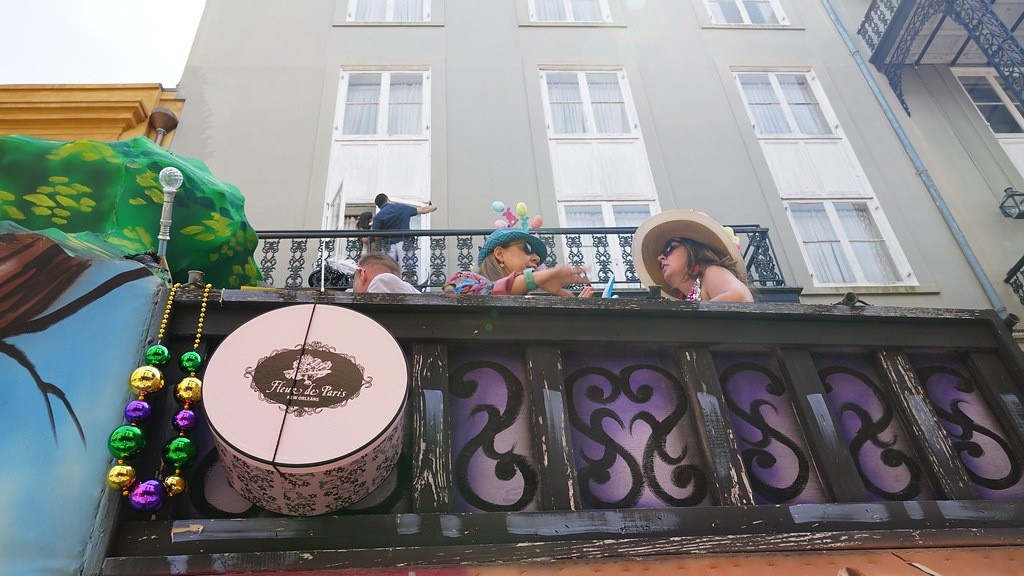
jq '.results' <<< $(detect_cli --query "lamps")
[998,186,1024,219]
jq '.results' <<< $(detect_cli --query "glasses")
[499,239,536,256]
[659,238,682,269]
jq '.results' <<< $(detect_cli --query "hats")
[478,227,547,267]
[632,210,747,302]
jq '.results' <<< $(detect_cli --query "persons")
[632,211,757,303]
[444,228,595,298]
[351,253,422,293]
[357,211,376,257]
[372,193,438,281]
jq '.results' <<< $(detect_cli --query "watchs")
[523,266,539,291]
